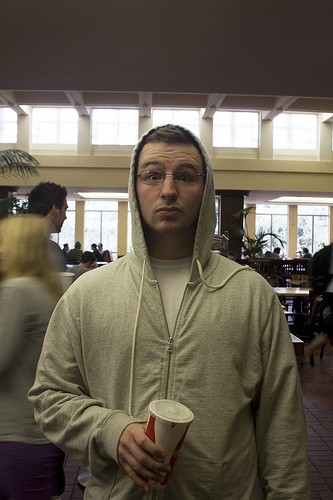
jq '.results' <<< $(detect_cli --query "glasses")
[135,170,205,185]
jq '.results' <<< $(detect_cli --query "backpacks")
[308,244,332,294]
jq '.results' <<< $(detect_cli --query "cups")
[58,271,77,293]
[137,399,195,489]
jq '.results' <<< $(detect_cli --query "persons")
[304,241,333,360]
[62,243,69,258]
[273,247,281,260]
[302,248,311,258]
[91,244,103,262]
[0,213,65,500]
[97,243,103,255]
[68,241,83,262]
[27,125,311,500]
[65,251,96,282]
[227,251,236,261]
[97,250,112,267]
[26,181,68,273]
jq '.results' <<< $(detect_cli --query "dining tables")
[271,287,313,334]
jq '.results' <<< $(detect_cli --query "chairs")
[243,258,328,367]
